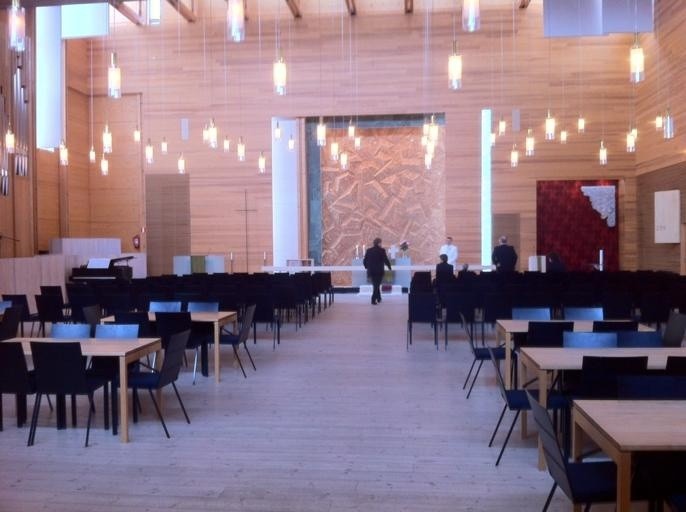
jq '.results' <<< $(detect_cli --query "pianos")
[68,256,134,284]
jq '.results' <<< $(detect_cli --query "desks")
[566,397,686,511]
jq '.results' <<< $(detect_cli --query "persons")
[546,251,561,273]
[435,254,454,283]
[456,263,469,281]
[362,237,391,306]
[438,235,458,272]
[491,235,518,272]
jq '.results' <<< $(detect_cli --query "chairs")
[404,266,686,404]
[527,390,659,510]
